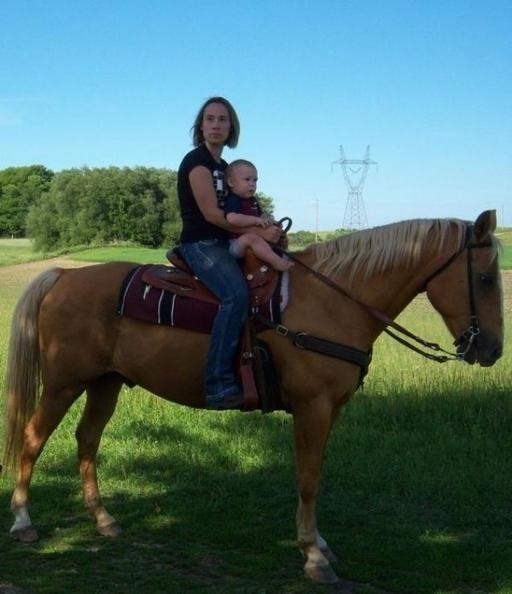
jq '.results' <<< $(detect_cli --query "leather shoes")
[206,385,262,410]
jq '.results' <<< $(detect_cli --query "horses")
[1,209,505,584]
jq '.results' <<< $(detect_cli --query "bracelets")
[255,216,260,226]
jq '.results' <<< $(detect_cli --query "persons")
[223,159,295,273]
[176,96,290,412]
